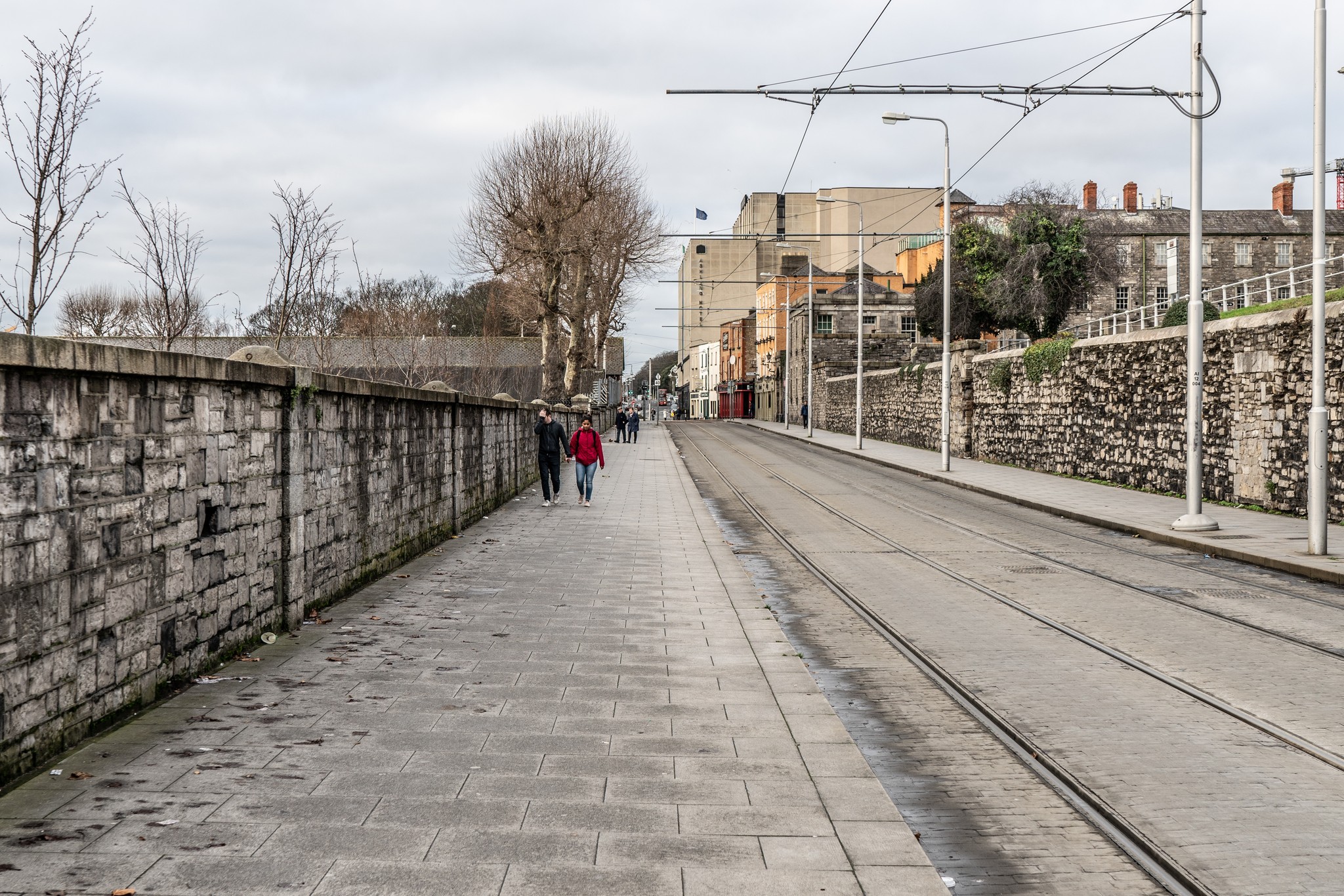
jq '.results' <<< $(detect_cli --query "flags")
[682,245,685,253]
[696,208,707,220]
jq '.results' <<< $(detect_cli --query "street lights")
[759,270,790,430]
[668,373,678,397]
[443,324,456,336]
[521,320,538,337]
[881,108,955,475]
[774,241,813,439]
[815,195,864,453]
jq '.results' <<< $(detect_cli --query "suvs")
[667,397,673,403]
[669,395,674,399]
[622,403,631,411]
[673,395,679,400]
[671,400,677,405]
[666,394,671,397]
[635,404,643,410]
[641,395,648,401]
[669,407,678,413]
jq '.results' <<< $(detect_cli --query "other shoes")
[614,440,619,443]
[626,441,630,443]
[633,441,636,444]
[752,417,753,419]
[623,441,626,443]
[806,425,808,428]
[804,427,805,429]
[748,418,751,419]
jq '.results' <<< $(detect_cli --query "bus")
[658,389,668,406]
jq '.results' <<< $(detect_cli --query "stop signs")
[631,399,636,403]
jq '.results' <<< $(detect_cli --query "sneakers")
[584,501,590,507]
[577,493,585,504]
[553,492,560,504]
[541,499,550,506]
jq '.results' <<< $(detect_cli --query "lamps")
[732,322,741,325]
[766,351,775,366]
[749,359,758,369]
[761,355,769,368]
[780,303,791,307]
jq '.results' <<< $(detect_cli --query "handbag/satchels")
[667,415,667,417]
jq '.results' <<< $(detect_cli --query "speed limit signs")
[654,380,661,386]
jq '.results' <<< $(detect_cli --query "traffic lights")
[648,395,650,398]
[631,391,633,393]
[628,391,630,394]
[647,390,649,392]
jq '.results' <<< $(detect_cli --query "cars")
[670,405,677,408]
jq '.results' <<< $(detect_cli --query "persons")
[663,409,668,420]
[676,407,682,420]
[651,408,656,421]
[614,406,627,443]
[749,400,754,419]
[534,407,571,507]
[800,400,808,429]
[623,407,640,443]
[565,416,605,507]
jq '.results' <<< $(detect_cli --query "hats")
[617,406,622,410]
